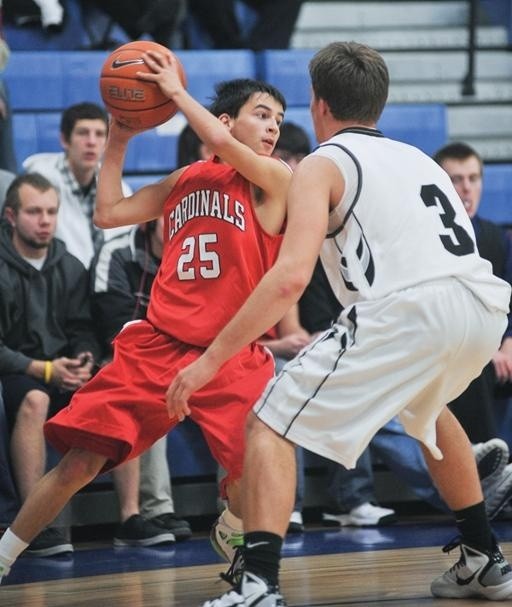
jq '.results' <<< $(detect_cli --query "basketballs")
[100,40,185,129]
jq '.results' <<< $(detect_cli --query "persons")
[165,41,511,605]
[265,120,511,533]
[0,105,227,558]
[1,51,292,581]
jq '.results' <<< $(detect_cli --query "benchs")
[6,50,510,525]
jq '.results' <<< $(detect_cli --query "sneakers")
[482,463,512,522]
[148,513,192,542]
[471,437,510,489]
[287,511,305,533]
[112,514,176,547]
[209,515,245,588]
[201,566,287,607]
[321,501,398,527]
[430,530,512,601]
[24,527,75,558]
